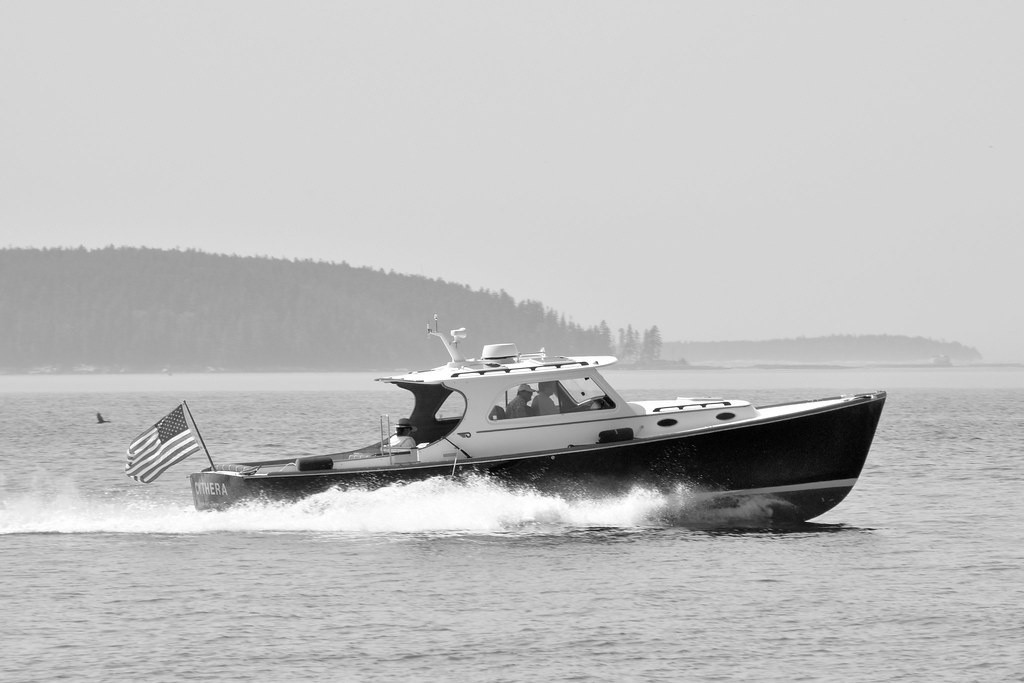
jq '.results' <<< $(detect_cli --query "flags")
[126,405,200,483]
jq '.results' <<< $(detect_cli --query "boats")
[125,313,888,533]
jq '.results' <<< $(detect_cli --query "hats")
[518,384,536,392]
[390,417,418,433]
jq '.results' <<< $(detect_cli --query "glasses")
[406,427,413,431]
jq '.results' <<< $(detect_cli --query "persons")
[390,418,417,447]
[531,381,558,415]
[506,384,534,418]
